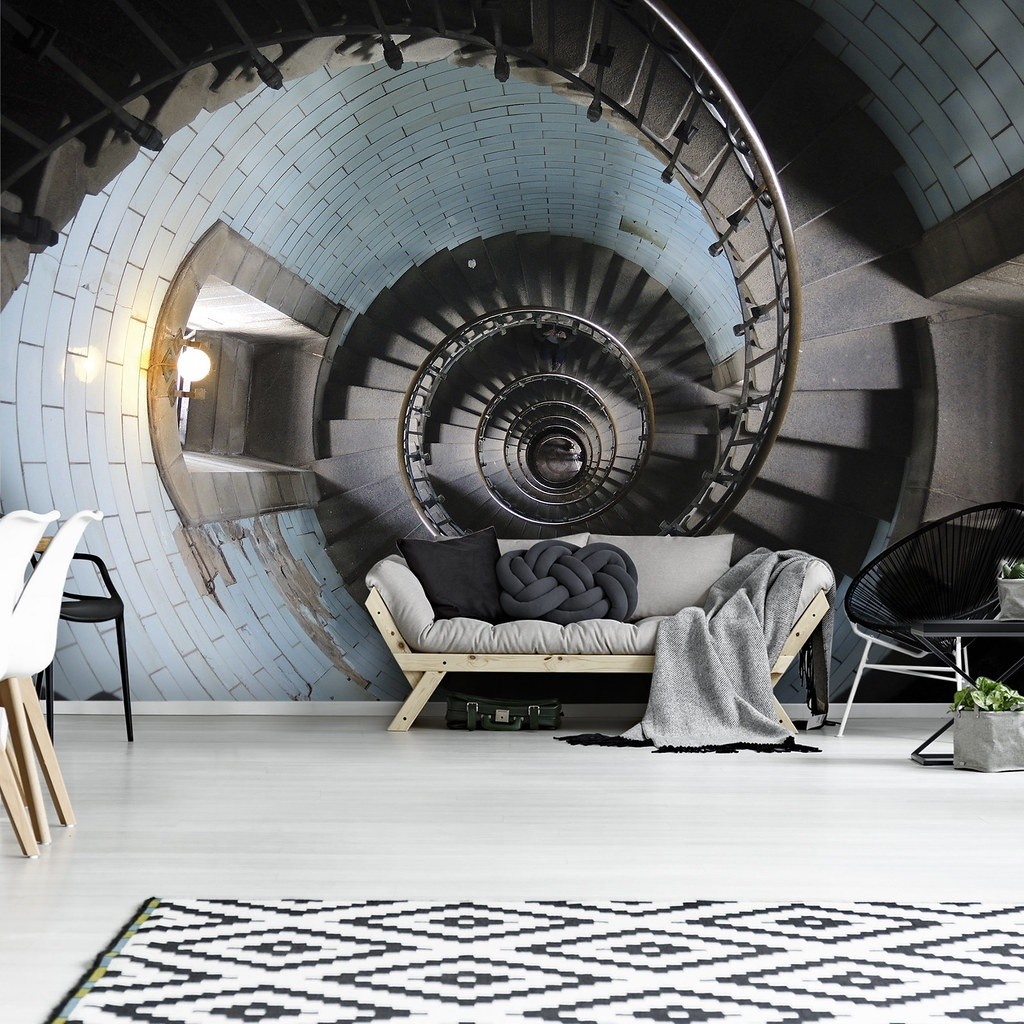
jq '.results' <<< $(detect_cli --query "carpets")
[41,896,1024,1024]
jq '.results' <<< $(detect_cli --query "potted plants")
[946,677,1024,773]
[994,558,1024,621]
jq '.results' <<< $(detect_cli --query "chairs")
[31,550,133,746]
[836,501,1024,736]
[12,509,104,828]
[0,510,60,860]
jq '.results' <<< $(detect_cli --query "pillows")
[433,533,590,557]
[588,534,734,620]
[395,526,511,626]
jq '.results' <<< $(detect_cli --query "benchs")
[365,533,829,732]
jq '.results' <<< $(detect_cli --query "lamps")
[159,328,212,406]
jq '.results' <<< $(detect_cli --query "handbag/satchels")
[445,688,562,730]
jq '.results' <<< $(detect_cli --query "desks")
[910,619,1024,765]
